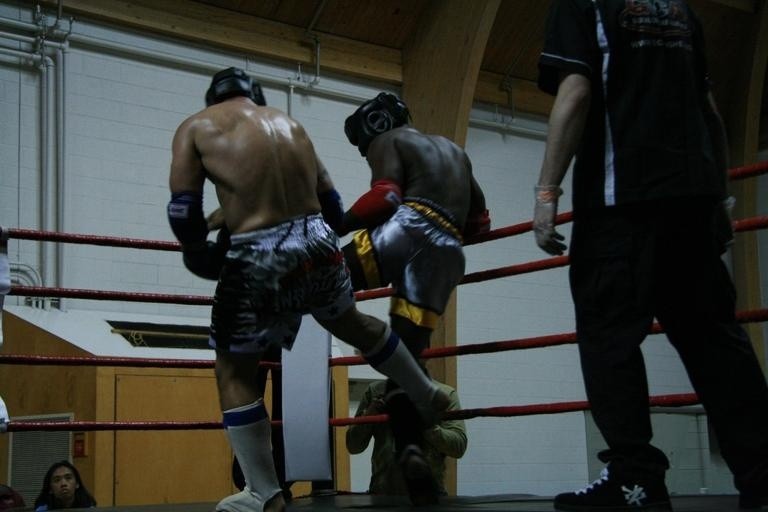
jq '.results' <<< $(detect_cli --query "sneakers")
[552,458,673,512]
[213,485,287,511]
[422,382,454,436]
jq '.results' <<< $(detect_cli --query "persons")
[0,482,24,510]
[530,0,766,510]
[166,67,452,512]
[332,90,492,509]
[345,338,467,496]
[33,461,97,511]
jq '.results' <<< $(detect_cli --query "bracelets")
[533,181,562,206]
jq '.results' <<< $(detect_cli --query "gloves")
[180,241,223,279]
[533,183,567,256]
[723,195,737,248]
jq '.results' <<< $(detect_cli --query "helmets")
[204,67,267,107]
[344,91,410,158]
